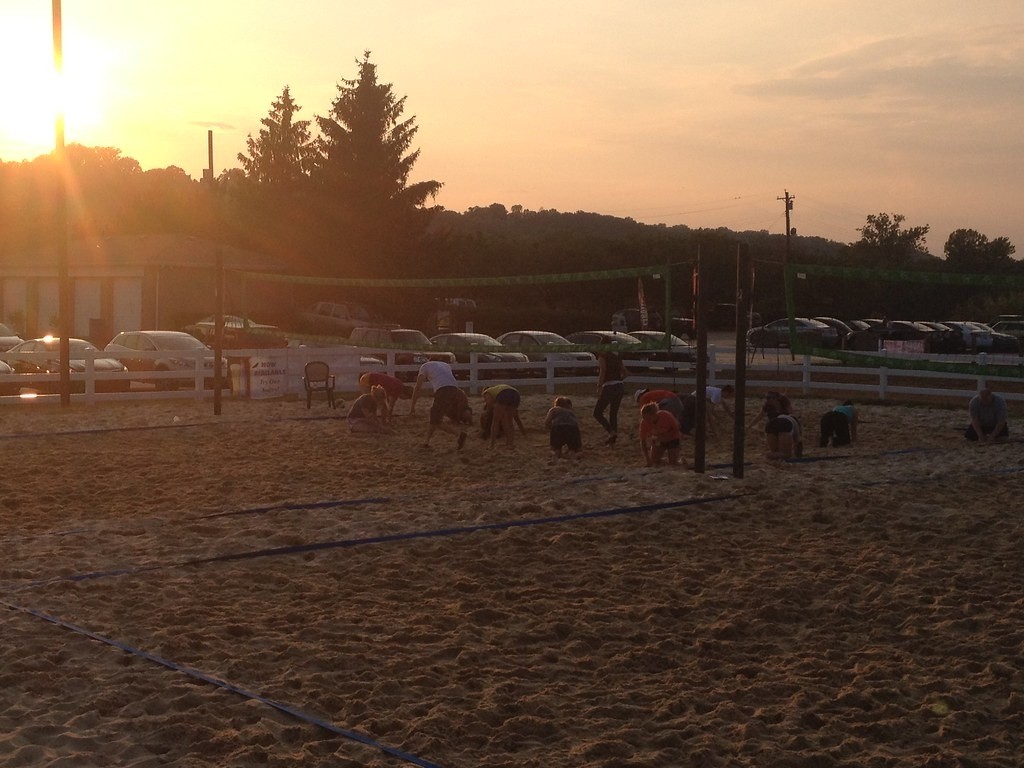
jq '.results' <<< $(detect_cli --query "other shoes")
[604,434,618,444]
[457,432,467,450]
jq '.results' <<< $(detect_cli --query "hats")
[633,388,646,402]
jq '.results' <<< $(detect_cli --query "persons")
[408,358,472,450]
[545,396,582,459]
[481,384,530,451]
[964,388,1009,444]
[748,391,799,458]
[347,372,413,436]
[593,351,630,446]
[818,400,858,448]
[630,384,736,467]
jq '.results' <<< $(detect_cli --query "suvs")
[347,324,457,381]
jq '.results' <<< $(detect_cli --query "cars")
[746,314,1024,353]
[429,329,698,379]
[0,321,228,395]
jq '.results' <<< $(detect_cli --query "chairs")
[302,361,337,409]
